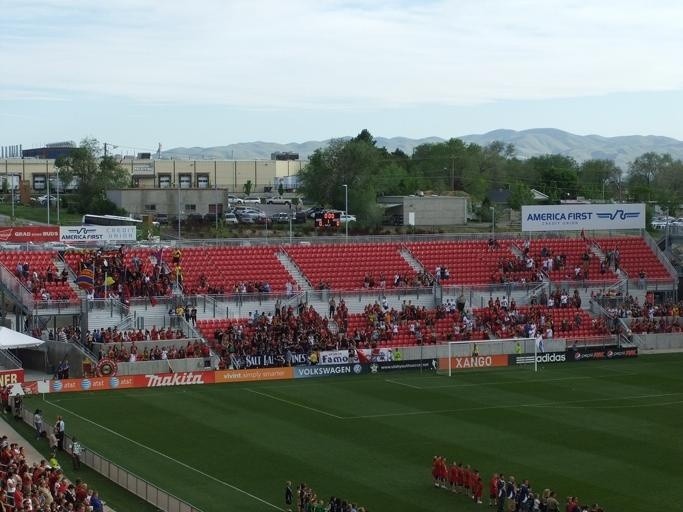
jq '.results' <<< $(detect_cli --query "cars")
[15,195,62,203]
[302,206,357,223]
[276,212,289,224]
[227,193,261,204]
[225,206,266,225]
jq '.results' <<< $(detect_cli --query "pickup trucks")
[265,196,292,205]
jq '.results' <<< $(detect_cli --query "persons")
[281,480,367,511]
[12,392,22,418]
[183,276,683,368]
[13,246,184,371]
[199,275,209,288]
[484,235,646,284]
[356,263,452,289]
[33,408,43,440]
[0,426,104,511]
[53,415,65,450]
[429,454,605,512]
[0,385,10,414]
